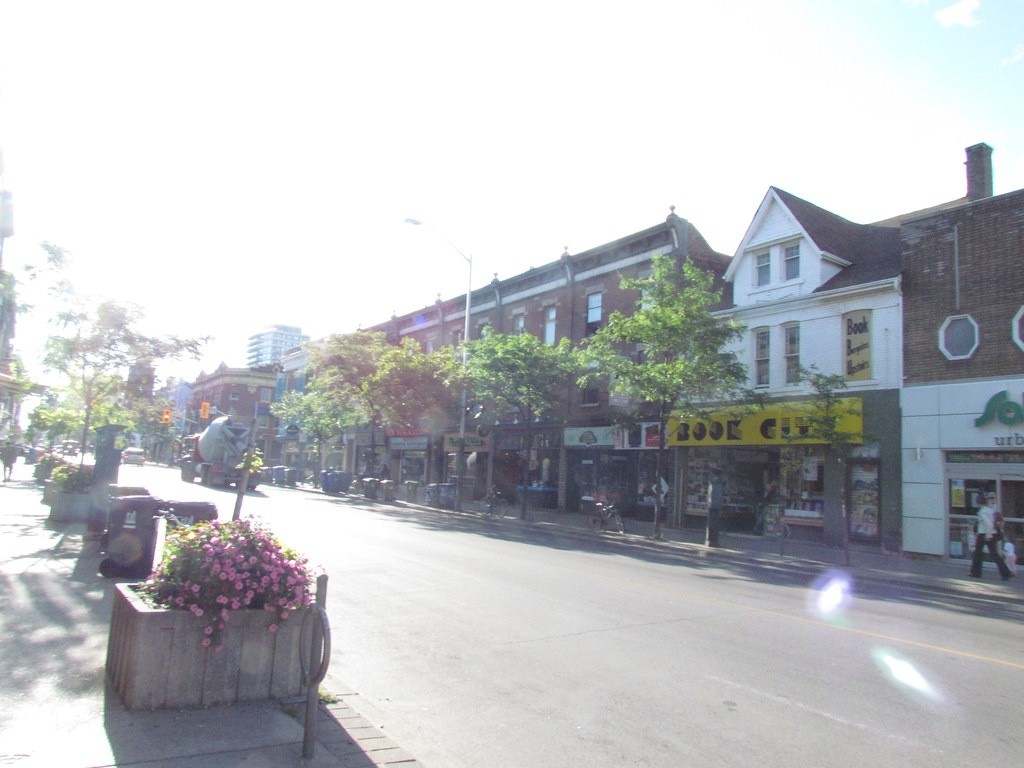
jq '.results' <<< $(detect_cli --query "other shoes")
[968,572,980,578]
[1001,572,1015,580]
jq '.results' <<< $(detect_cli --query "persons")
[0,442,16,481]
[969,495,1016,580]
[381,463,391,478]
[754,477,777,534]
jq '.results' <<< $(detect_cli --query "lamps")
[494,417,540,425]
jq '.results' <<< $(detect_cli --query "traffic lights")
[162,409,171,424]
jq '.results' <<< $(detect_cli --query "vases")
[39,477,92,523]
[104,582,311,712]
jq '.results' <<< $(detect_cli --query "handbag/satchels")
[993,512,1010,533]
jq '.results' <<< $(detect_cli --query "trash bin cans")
[97,487,153,547]
[405,481,419,502]
[363,478,381,499]
[381,481,398,501]
[262,465,297,488]
[320,470,350,495]
[97,495,218,577]
[425,483,456,510]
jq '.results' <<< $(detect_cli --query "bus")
[62,439,80,456]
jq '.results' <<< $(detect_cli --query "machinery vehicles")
[179,414,260,491]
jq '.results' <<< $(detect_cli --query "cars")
[121,446,146,468]
[56,445,63,454]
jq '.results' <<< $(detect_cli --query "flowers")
[50,463,97,495]
[133,514,326,656]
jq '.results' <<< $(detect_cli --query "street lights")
[400,217,473,512]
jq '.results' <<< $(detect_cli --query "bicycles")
[478,483,509,521]
[760,503,791,541]
[588,498,625,535]
